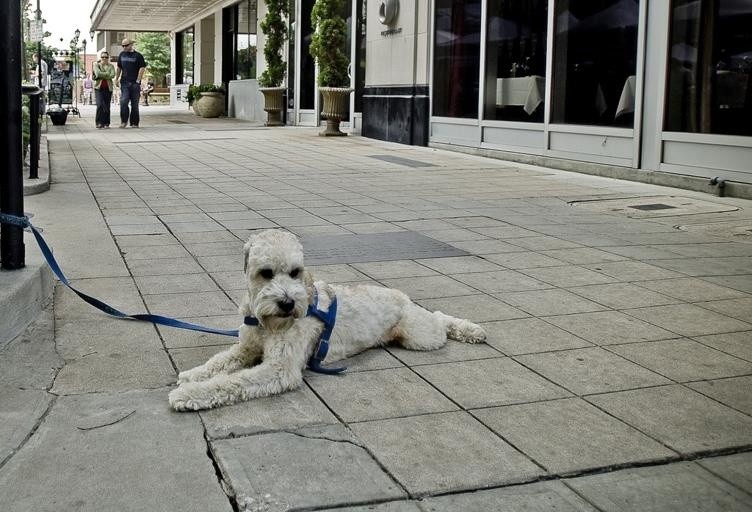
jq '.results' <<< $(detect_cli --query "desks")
[493,74,545,115]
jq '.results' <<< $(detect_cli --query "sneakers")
[96,122,110,129]
[119,121,139,129]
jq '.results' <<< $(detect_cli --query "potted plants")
[45,107,70,125]
[256,0,358,138]
[186,84,229,119]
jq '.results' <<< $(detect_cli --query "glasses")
[122,44,130,48]
[101,57,109,59]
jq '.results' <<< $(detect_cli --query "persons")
[144,77,154,106]
[115,38,146,129]
[84,74,92,105]
[92,52,115,128]
[30,54,48,115]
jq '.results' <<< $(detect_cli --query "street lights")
[74,28,81,114]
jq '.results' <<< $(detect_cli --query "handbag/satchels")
[92,70,100,80]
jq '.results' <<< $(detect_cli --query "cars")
[715,51,752,114]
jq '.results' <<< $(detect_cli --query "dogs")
[166,230,487,413]
